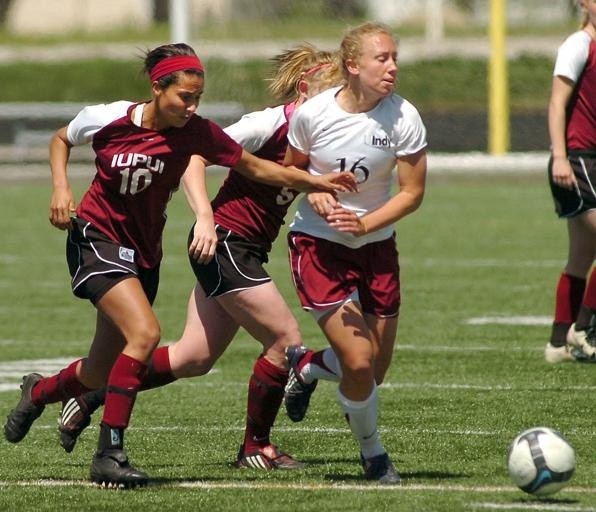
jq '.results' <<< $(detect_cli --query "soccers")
[507,425,577,497]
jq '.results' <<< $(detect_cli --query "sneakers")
[90,449,149,487]
[568,324,596,358]
[5,373,44,444]
[358,452,402,485]
[236,445,306,474]
[285,345,318,423]
[546,341,581,363]
[58,396,90,454]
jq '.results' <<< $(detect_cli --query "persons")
[281,21,430,484]
[4,41,360,484]
[542,1,596,364]
[55,40,344,471]
